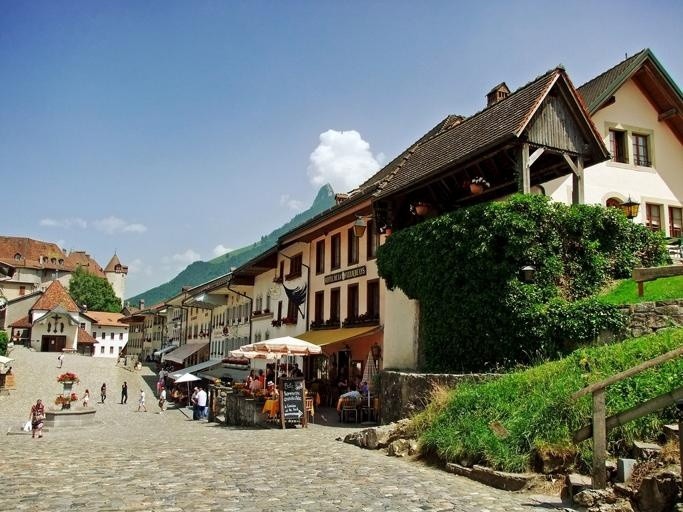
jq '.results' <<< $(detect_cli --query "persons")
[56,352,65,366]
[28,399,45,438]
[79,356,366,420]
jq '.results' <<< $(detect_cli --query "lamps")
[618,194,640,219]
[351,212,381,238]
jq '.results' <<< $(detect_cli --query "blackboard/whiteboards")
[280,377,308,420]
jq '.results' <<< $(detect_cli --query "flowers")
[54,371,80,410]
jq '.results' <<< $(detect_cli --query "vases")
[379,176,490,233]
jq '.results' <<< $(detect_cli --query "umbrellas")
[241,336,321,377]
[239,344,306,389]
[361,351,378,406]
[227,349,280,380]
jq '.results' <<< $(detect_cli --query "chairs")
[305,382,380,425]
